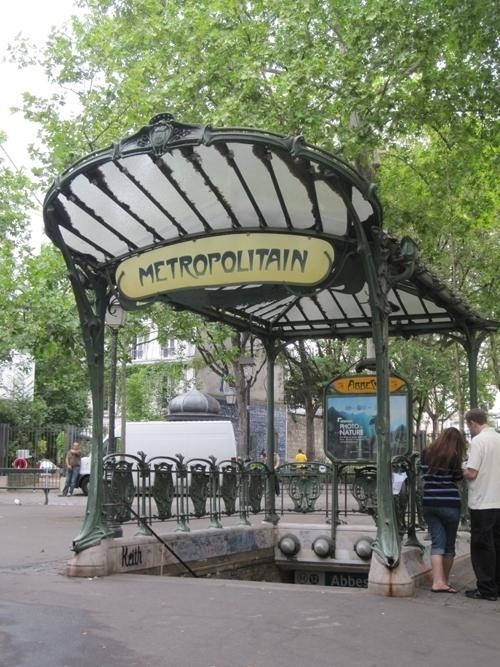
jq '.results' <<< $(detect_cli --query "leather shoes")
[464,590,496,601]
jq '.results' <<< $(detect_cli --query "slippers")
[431,585,457,593]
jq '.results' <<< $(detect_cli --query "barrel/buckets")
[12,458,28,469]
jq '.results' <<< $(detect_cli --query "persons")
[260,448,282,470]
[458,407,500,601]
[57,441,82,497]
[420,425,466,593]
[295,448,308,473]
[39,454,57,468]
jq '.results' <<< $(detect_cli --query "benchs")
[0,468,60,505]
